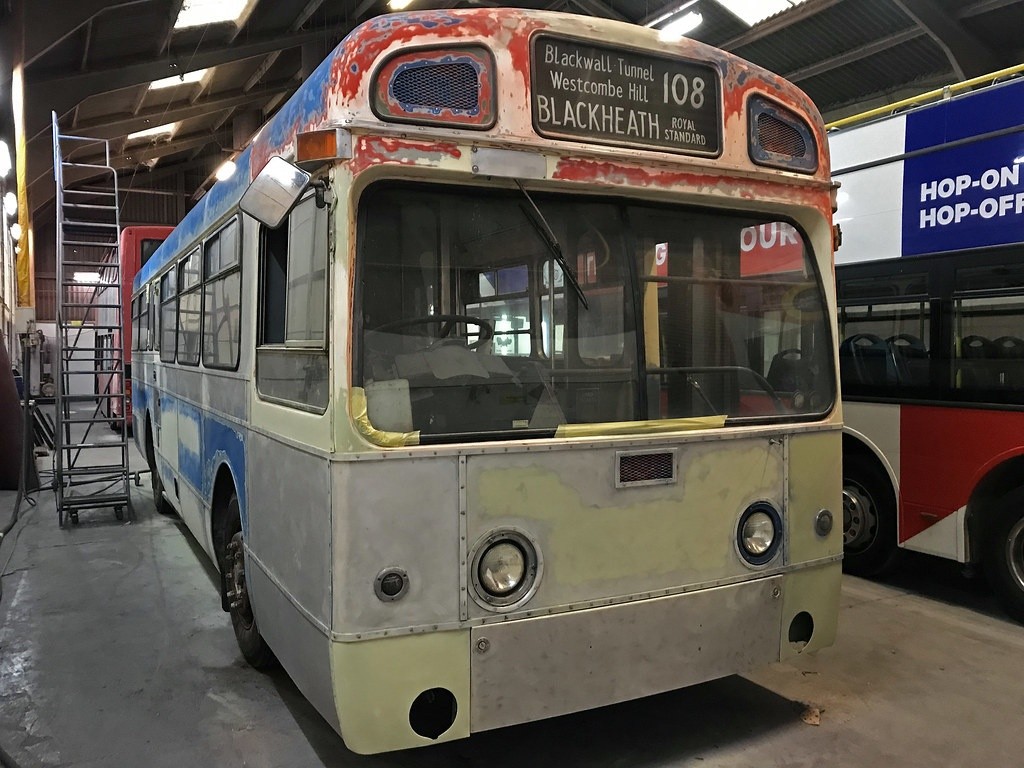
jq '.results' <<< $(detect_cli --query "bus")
[93,225,182,438]
[130,6,845,757]
[563,242,1023,624]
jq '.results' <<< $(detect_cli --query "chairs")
[766,349,802,392]
[891,334,930,399]
[960,335,1024,405]
[850,334,898,398]
